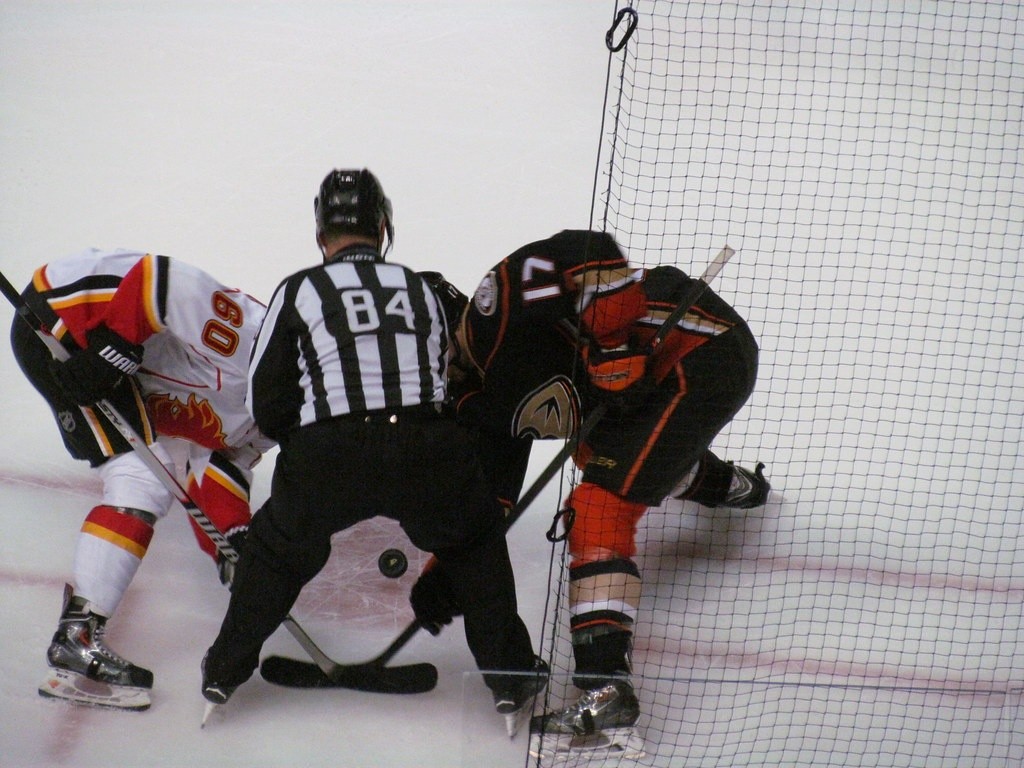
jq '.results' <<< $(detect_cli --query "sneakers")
[717,460,786,509]
[38,583,153,712]
[529,638,646,760]
[492,655,548,742]
[201,647,240,730]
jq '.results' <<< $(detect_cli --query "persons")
[9,247,278,711]
[414,230,787,761]
[199,167,549,739]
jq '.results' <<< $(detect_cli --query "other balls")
[377,549,408,578]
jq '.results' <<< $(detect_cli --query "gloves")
[410,557,478,637]
[582,336,647,391]
[216,525,250,594]
[52,323,145,406]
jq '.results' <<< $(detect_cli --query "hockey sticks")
[0,244,737,694]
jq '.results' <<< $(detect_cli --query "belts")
[352,401,452,425]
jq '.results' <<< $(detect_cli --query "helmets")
[416,271,468,324]
[314,168,393,245]
